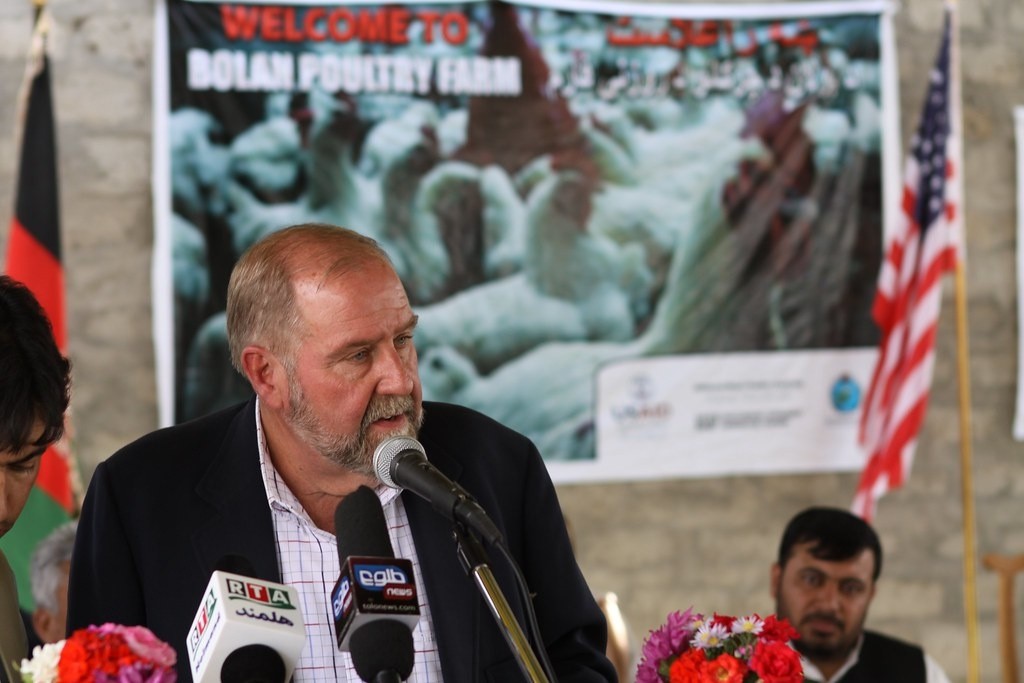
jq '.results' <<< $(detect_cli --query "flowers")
[12,623,177,683]
[635,605,804,683]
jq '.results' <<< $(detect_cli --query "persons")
[0,273,71,683]
[65,222,618,683]
[769,507,950,683]
[31,520,78,641]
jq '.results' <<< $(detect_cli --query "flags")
[849,10,963,524]
[0,8,78,615]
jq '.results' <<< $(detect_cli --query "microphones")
[186,554,307,683]
[330,484,421,683]
[373,435,505,548]
